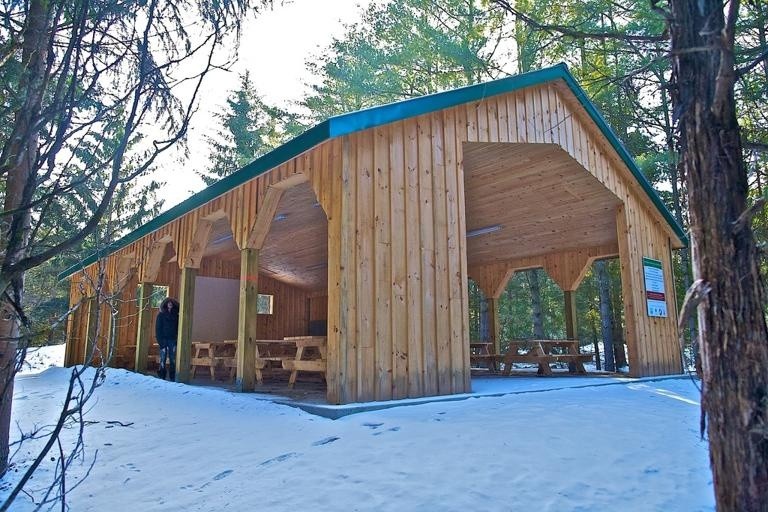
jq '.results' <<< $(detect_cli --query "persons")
[155,298,178,381]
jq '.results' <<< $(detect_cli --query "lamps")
[465,223,505,241]
[210,234,235,246]
[274,213,286,223]
[309,202,320,209]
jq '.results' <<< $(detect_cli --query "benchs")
[117,333,328,390]
[470,337,593,381]
